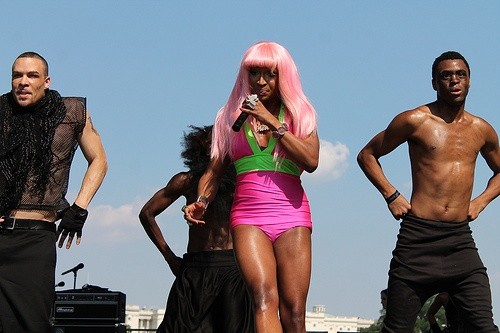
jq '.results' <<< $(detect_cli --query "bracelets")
[386,190,400,204]
[272,120,290,139]
[197,195,210,209]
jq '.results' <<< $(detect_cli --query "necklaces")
[256,118,271,134]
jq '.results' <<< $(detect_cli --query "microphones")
[62,263,84,275]
[232,109,251,132]
[55,281,65,287]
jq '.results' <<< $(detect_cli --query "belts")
[0,216,57,232]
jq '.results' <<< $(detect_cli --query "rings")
[181,205,190,213]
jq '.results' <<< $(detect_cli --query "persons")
[138,125,256,333]
[357,51,500,333]
[0,51,108,333]
[182,41,319,332]
